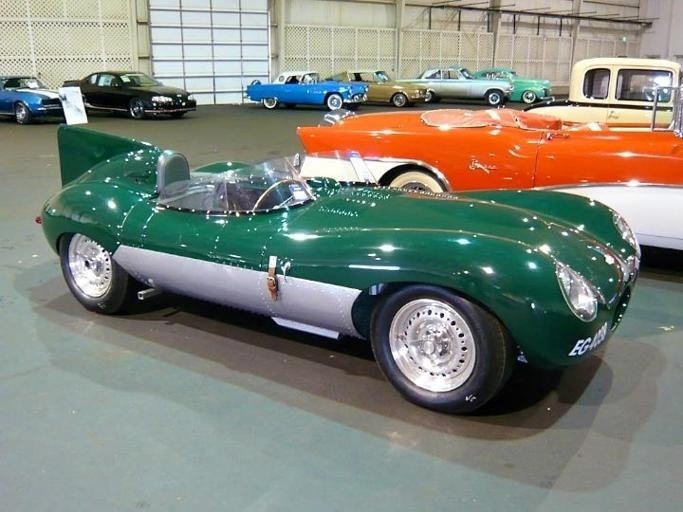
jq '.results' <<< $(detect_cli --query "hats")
[291,82,683,271]
[0,74,88,124]
[57,67,200,122]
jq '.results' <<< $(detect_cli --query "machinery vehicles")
[242,70,370,116]
[26,121,648,430]
